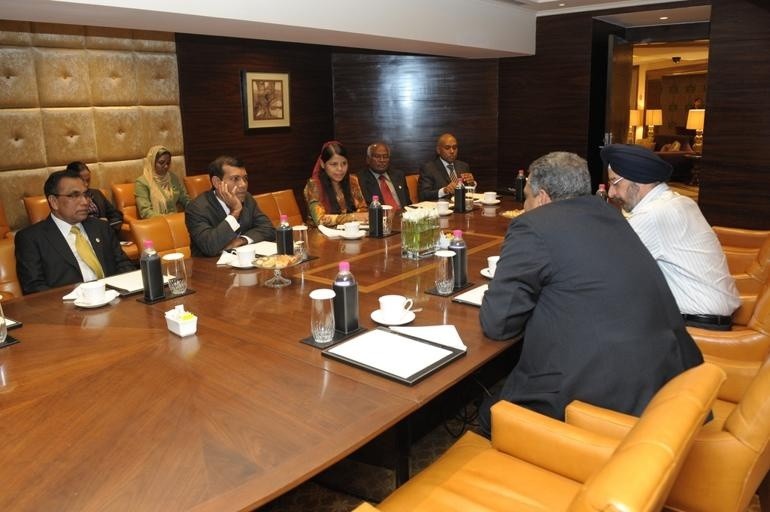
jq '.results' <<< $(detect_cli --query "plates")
[371,309,415,326]
[438,210,454,216]
[480,268,494,279]
[338,234,365,240]
[231,262,255,270]
[74,292,116,308]
[480,200,501,206]
[481,205,501,209]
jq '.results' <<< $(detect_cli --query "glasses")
[52,191,93,199]
[607,177,623,188]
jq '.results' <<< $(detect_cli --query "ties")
[70,226,104,279]
[378,176,399,211]
[447,165,458,187]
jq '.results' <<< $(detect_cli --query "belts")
[682,314,731,325]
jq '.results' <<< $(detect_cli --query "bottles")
[515,170,526,203]
[596,184,608,204]
[141,239,166,302]
[276,214,293,255]
[454,178,465,213]
[448,230,468,291]
[333,260,358,335]
[369,195,382,237]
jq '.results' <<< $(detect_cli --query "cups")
[481,208,496,218]
[341,221,361,236]
[437,202,449,213]
[439,218,449,229]
[0,302,7,343]
[382,205,394,236]
[434,250,456,295]
[233,272,258,287]
[161,252,188,294]
[463,213,476,234]
[378,295,414,319]
[484,191,499,202]
[340,244,361,255]
[231,248,256,265]
[78,281,106,303]
[309,287,337,342]
[81,314,110,330]
[486,255,501,275]
[292,225,309,260]
[0,365,11,387]
[465,185,475,213]
[605,132,613,145]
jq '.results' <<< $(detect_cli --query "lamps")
[685,109,705,153]
[628,110,644,144]
[646,109,663,143]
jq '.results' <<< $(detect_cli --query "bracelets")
[351,212,355,222]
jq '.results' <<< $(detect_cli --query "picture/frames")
[239,66,294,137]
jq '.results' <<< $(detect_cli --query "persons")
[358,141,413,212]
[600,144,743,331]
[134,147,192,219]
[687,98,705,109]
[303,141,370,227]
[67,161,124,235]
[185,156,277,261]
[476,150,714,440]
[418,134,479,201]
[15,170,138,295]
